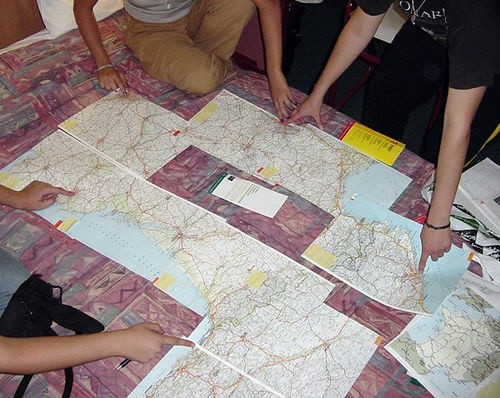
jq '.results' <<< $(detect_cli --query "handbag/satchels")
[0,274,105,398]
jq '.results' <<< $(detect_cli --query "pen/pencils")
[114,358,133,371]
[208,172,227,193]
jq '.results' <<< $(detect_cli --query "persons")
[0,180,195,374]
[73,0,298,123]
[284,0,497,272]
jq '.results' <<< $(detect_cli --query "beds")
[1,0,500,398]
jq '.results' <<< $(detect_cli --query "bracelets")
[425,219,450,230]
[97,64,114,71]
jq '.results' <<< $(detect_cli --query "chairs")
[326,0,446,129]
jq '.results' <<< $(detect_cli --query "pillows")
[37,0,127,40]
